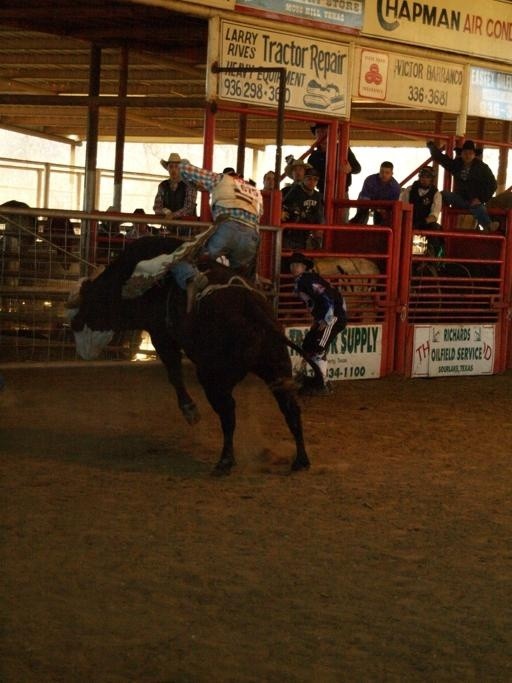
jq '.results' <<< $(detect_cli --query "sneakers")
[297,381,335,395]
[486,221,500,233]
[186,273,208,315]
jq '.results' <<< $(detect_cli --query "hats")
[160,153,183,170]
[285,159,312,179]
[309,123,326,137]
[454,140,483,157]
[283,252,314,272]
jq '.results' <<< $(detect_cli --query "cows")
[409,262,500,323]
[308,257,381,324]
[62,235,326,477]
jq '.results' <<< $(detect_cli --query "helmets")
[304,168,321,177]
[419,166,436,178]
